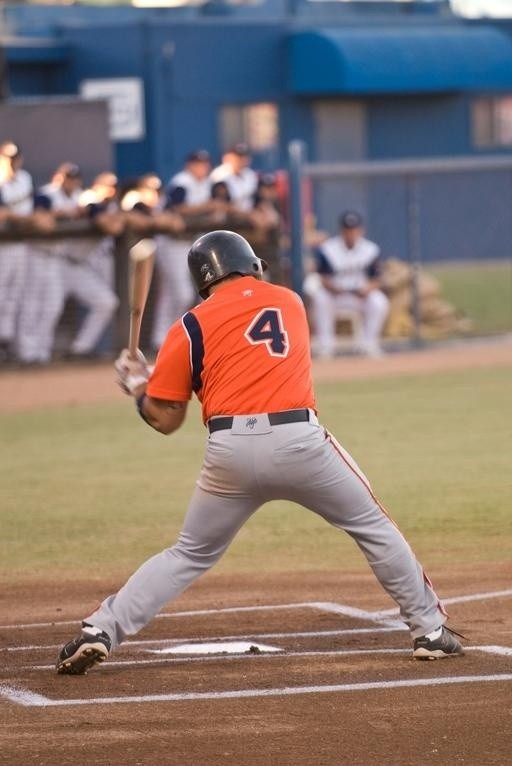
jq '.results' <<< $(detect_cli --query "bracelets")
[127,375,151,396]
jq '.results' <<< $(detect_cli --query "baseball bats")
[129,240,155,362]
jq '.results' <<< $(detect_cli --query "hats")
[2,141,294,214]
[338,210,362,230]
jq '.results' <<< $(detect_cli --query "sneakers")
[318,338,336,359]
[354,338,383,358]
[413,624,465,661]
[55,624,112,674]
[0,333,103,369]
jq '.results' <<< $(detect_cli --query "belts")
[207,408,309,434]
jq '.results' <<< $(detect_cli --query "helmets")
[188,229,269,300]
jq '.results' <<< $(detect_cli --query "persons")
[303,209,392,364]
[1,138,292,370]
[55,228,467,676]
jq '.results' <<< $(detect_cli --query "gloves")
[115,346,154,395]
